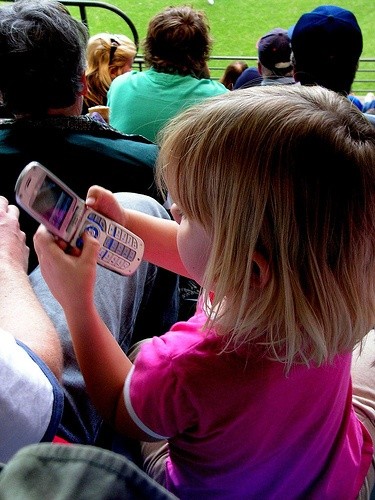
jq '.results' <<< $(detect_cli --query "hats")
[287,6,363,66]
[258,29,294,73]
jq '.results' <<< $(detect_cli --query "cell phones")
[14,161,146,276]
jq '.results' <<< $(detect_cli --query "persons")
[107,6,231,143]
[288,5,375,131]
[80,33,136,125]
[33,84,375,500]
[0,192,179,464]
[220,27,296,92]
[0,0,200,347]
